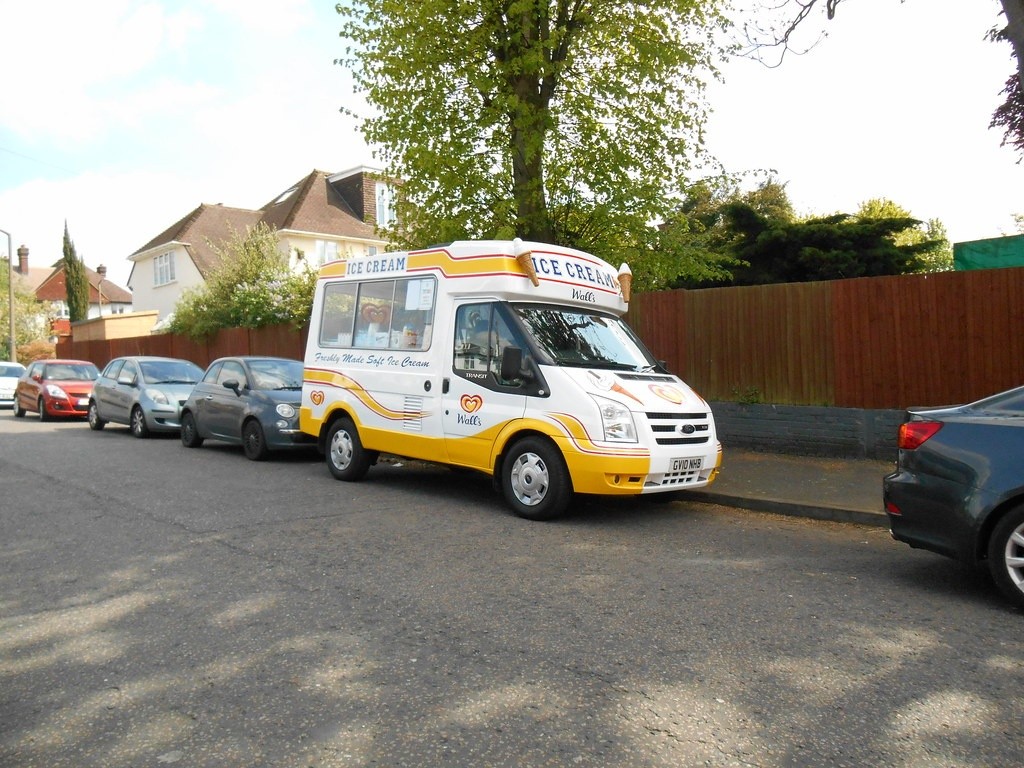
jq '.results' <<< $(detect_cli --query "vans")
[299,239,724,519]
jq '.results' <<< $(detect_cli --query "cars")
[88,356,204,438]
[12,360,102,422]
[0,362,27,409]
[881,385,1024,606]
[179,354,325,461]
